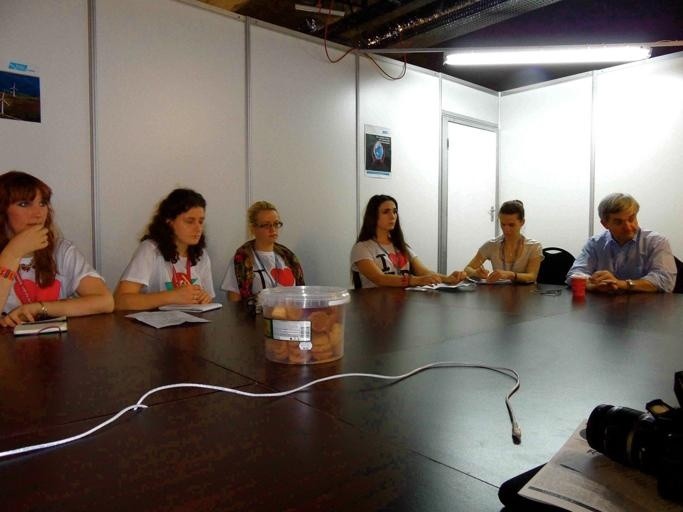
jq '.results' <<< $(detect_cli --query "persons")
[221,200,305,302]
[564,192,677,294]
[348,193,467,291]
[112,188,217,313]
[0,170,116,329]
[463,200,546,284]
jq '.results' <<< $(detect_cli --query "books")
[158,302,223,314]
[498,463,569,511]
[13,316,68,336]
[479,278,513,285]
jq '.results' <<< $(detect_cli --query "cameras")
[586,371,683,503]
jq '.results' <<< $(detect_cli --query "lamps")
[444,43,653,65]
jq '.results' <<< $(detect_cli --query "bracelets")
[39,301,48,320]
[409,274,415,286]
[399,272,409,287]
[1,266,17,282]
[511,272,517,282]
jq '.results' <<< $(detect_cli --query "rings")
[193,295,196,300]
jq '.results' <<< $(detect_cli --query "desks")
[1,283,683,512]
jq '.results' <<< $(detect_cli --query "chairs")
[537,247,575,285]
[670,255,683,294]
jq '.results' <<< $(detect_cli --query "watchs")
[625,279,635,290]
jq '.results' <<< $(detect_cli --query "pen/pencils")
[179,272,192,286]
[479,260,485,270]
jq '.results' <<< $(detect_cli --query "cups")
[569,274,587,298]
[257,286,352,366]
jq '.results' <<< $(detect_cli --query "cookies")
[259,303,344,365]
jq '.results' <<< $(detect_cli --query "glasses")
[254,222,283,230]
